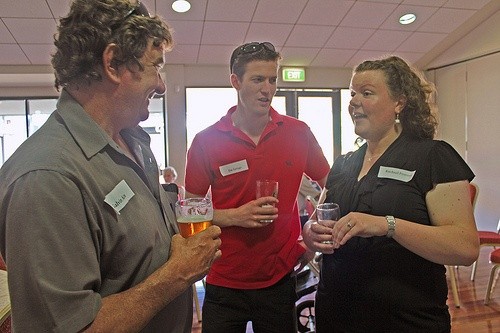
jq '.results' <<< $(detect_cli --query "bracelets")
[385,215,396,239]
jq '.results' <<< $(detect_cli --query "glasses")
[110,1,150,31]
[231,42,275,74]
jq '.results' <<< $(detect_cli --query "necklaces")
[364,143,389,163]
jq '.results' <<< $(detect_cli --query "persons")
[162,167,185,199]
[185,41,330,333]
[303,56,480,333]
[0,0,222,333]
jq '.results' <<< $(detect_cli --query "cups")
[255,179,279,222]
[316,203,341,244]
[175,198,213,240]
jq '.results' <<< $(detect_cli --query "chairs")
[448,183,500,308]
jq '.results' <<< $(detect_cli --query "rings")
[347,222,353,228]
